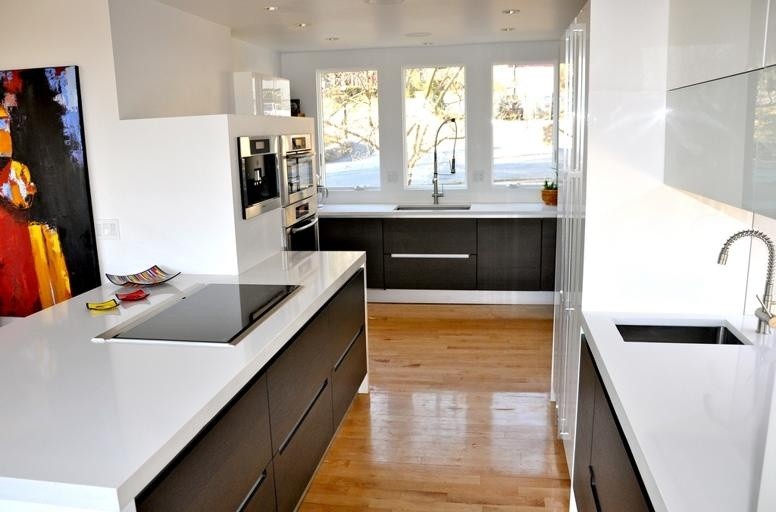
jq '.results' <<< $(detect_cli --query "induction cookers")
[94,281,305,346]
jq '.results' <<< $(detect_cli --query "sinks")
[613,316,754,346]
[395,204,472,211]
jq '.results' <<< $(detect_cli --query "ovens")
[279,194,320,251]
[280,133,320,207]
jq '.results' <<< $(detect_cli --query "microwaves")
[236,136,283,220]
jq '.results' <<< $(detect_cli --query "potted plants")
[542,181,558,206]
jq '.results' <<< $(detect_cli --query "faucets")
[717,228,775,335]
[431,117,458,204]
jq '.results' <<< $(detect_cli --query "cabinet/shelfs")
[383,218,477,290]
[662,0,775,220]
[542,218,556,291]
[0,252,370,512]
[478,219,542,291]
[572,310,775,511]
[319,218,383,289]
